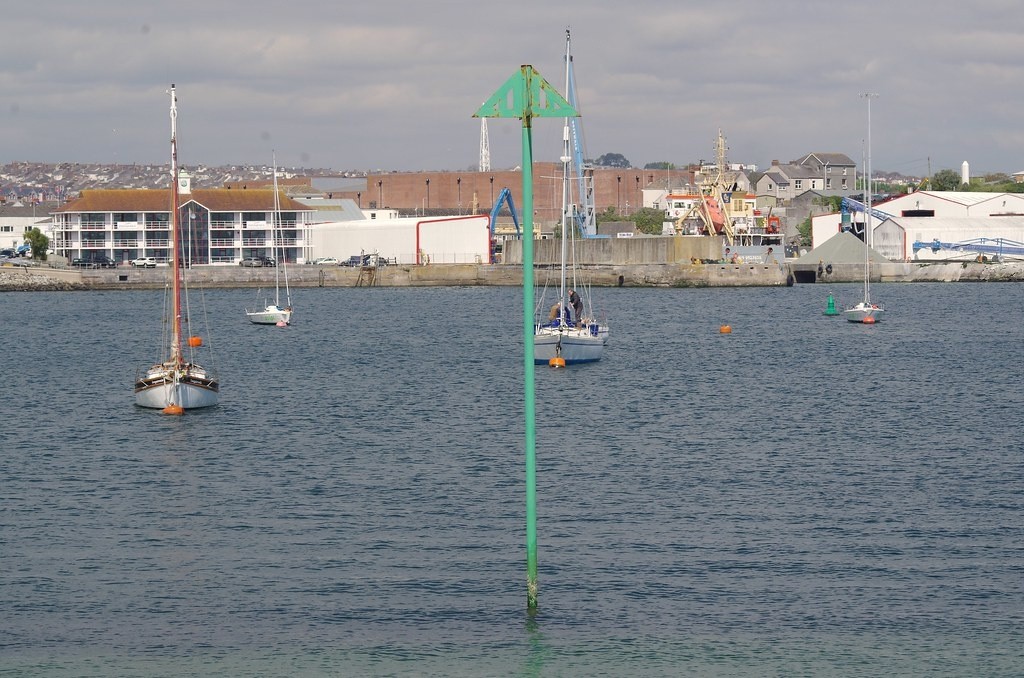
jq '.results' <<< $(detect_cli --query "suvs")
[130,256,157,269]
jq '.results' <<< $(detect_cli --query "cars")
[168,256,194,268]
[305,252,388,268]
[238,256,262,267]
[72,255,118,269]
[260,256,279,267]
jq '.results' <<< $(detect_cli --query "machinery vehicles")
[489,188,522,262]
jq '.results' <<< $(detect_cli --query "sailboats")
[533,25,609,366]
[842,139,886,324]
[132,84,222,410]
[243,148,296,325]
